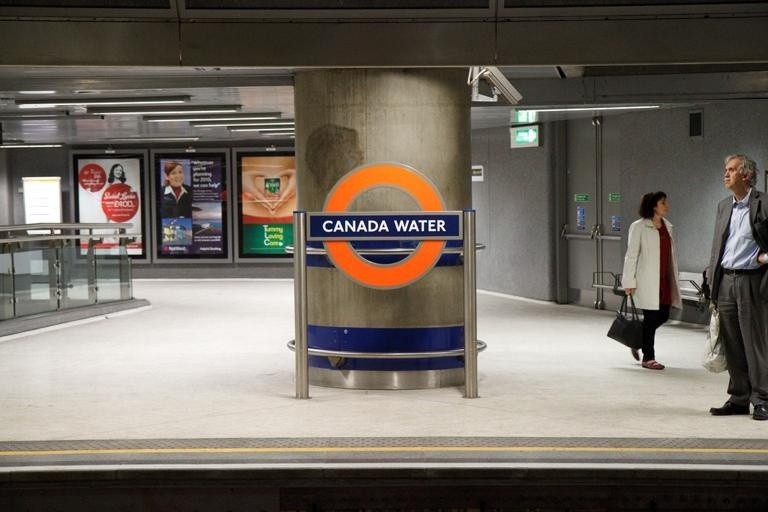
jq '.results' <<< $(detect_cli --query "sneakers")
[631,348,640,361]
[642,360,665,370]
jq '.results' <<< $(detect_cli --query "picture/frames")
[71,151,296,259]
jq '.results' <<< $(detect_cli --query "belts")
[721,269,763,275]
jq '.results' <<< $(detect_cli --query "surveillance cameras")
[481,66,523,106]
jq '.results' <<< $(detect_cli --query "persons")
[157,159,192,219]
[620,190,683,369]
[107,163,127,187]
[242,167,297,216]
[705,153,768,420]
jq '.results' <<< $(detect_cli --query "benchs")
[591,271,708,318]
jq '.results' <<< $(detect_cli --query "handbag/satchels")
[702,308,728,373]
[607,310,644,349]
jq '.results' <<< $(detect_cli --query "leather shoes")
[753,404,768,420]
[710,401,750,416]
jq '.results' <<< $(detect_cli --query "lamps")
[0,97,297,149]
